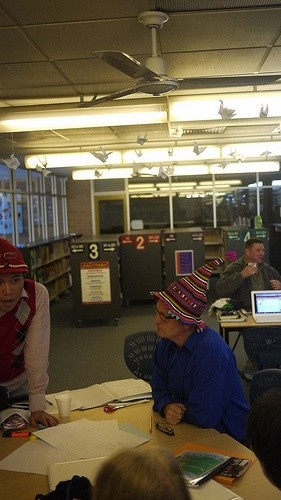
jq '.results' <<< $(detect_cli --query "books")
[68,377,153,410]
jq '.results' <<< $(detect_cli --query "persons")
[150,249,250,441]
[0,238,58,427]
[215,239,281,379]
[93,445,192,500]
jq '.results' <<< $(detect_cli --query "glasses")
[154,304,176,321]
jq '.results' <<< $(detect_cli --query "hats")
[0,237,29,274]
[148,251,237,333]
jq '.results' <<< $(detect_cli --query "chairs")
[242,326,281,489]
[124,330,161,382]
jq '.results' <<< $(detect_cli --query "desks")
[0,398,281,500]
[216,309,281,352]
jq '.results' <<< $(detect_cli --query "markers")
[3,431,30,437]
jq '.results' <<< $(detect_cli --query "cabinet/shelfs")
[204,241,223,276]
[17,233,82,302]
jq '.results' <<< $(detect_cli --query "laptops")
[251,290,281,323]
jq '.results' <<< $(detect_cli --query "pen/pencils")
[149,416,152,434]
[46,400,54,406]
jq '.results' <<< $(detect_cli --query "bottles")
[254,216,262,230]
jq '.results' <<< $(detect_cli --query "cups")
[248,263,257,274]
[55,398,72,417]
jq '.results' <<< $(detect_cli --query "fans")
[78,11,281,108]
